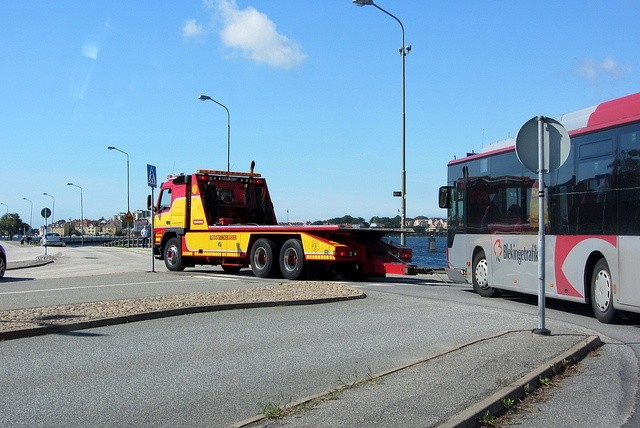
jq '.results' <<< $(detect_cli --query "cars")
[40,232,65,246]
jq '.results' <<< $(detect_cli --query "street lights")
[67,183,84,247]
[352,0,411,246]
[197,94,230,172]
[0,202,8,219]
[23,197,32,225]
[108,147,130,248]
[42,193,54,233]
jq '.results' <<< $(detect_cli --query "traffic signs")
[147,164,157,188]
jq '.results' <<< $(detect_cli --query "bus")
[438,92,639,323]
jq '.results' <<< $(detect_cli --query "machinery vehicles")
[147,161,415,279]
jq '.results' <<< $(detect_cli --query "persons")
[141,225,149,248]
